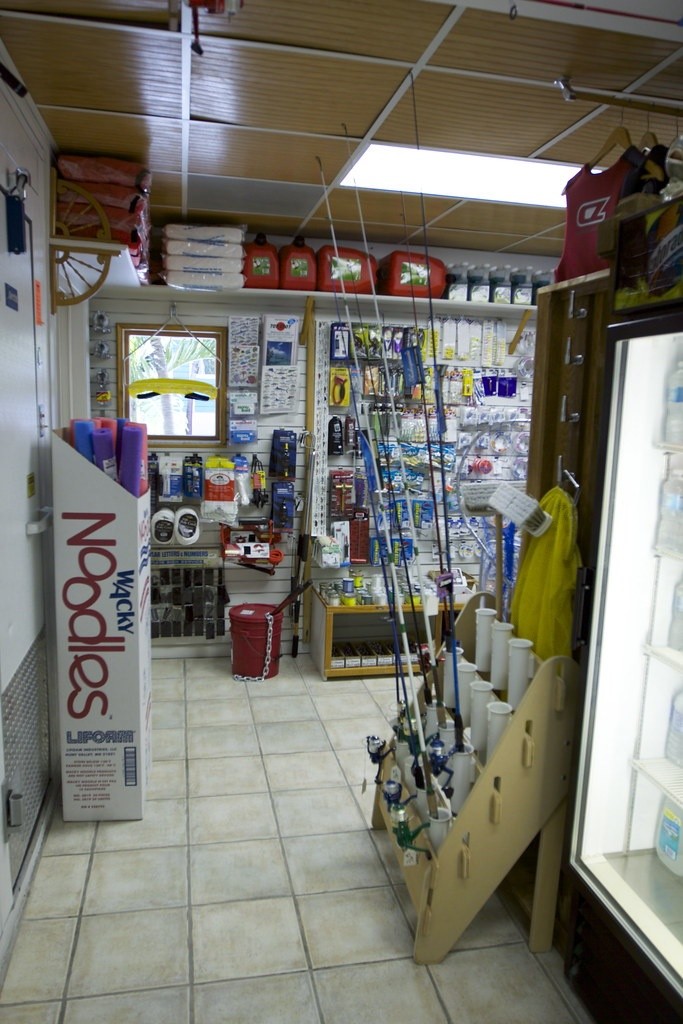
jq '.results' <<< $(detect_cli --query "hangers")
[560,127,665,196]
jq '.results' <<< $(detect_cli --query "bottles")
[244,233,551,304]
[655,797,683,878]
[661,361,683,446]
[665,692,682,768]
[654,468,683,555]
[667,571,683,651]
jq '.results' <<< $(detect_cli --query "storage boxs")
[50,427,149,823]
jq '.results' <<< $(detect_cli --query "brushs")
[487,481,554,537]
[458,480,526,623]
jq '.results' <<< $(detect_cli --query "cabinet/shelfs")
[371,591,578,964]
[310,583,463,681]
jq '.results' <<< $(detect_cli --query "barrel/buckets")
[230,603,283,682]
